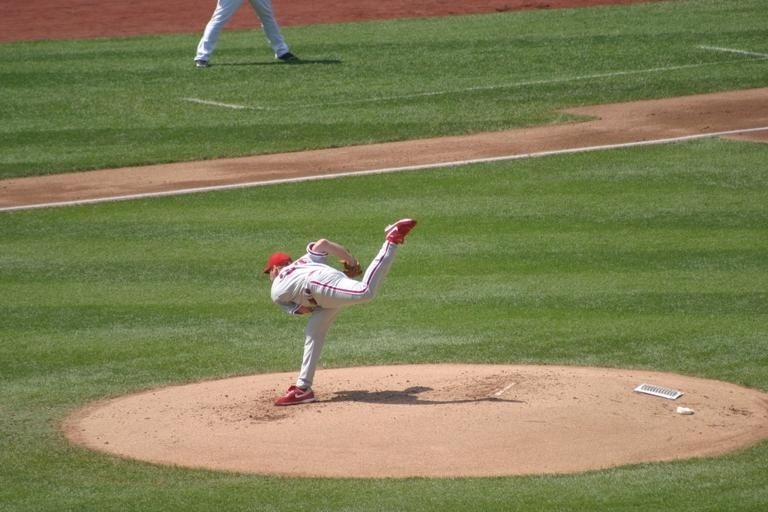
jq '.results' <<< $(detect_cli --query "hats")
[262,253,293,275]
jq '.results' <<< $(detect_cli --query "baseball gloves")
[340,261,362,278]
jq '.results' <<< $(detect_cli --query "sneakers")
[274,51,300,62]
[275,385,316,406]
[383,217,418,244]
[195,59,208,68]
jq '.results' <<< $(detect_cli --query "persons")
[263,219,418,406]
[194,0,298,68]
